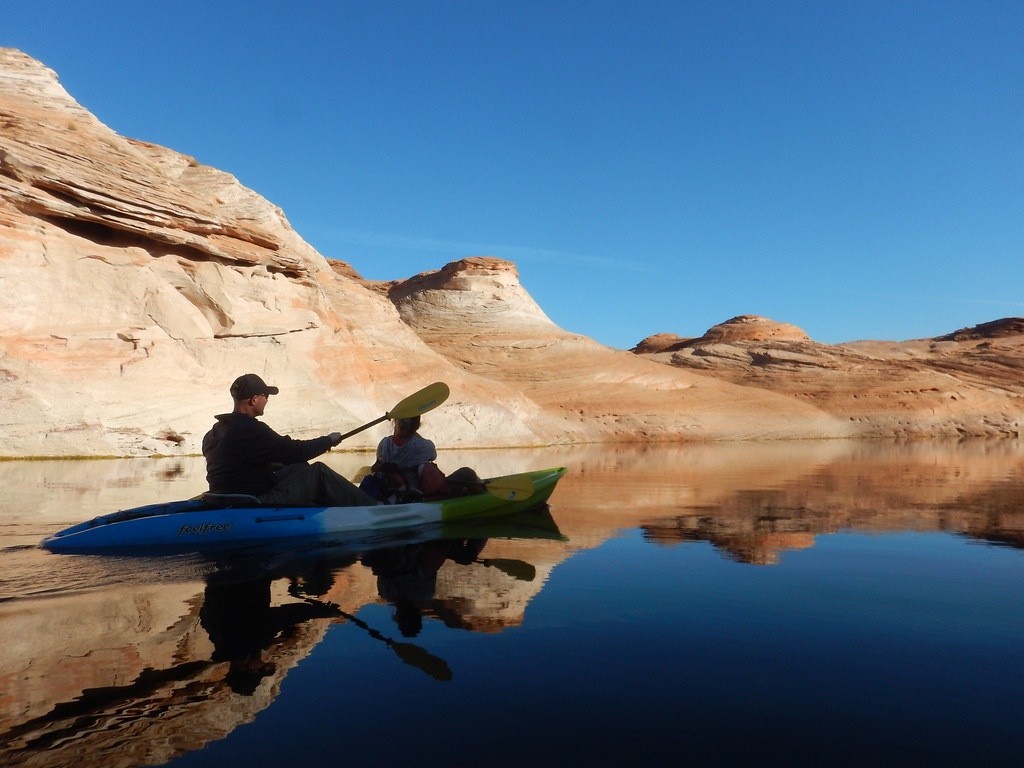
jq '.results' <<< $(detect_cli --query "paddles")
[446,476,534,500]
[473,558,536,582]
[330,382,450,447]
[290,593,453,682]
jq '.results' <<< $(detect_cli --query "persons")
[199,553,341,696]
[361,538,489,637]
[376,415,481,498]
[202,374,412,508]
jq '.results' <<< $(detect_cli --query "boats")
[39,465,569,552]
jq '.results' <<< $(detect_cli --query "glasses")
[261,394,268,399]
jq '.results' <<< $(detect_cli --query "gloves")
[328,432,341,447]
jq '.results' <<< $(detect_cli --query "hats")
[230,374,279,400]
[226,661,275,696]
